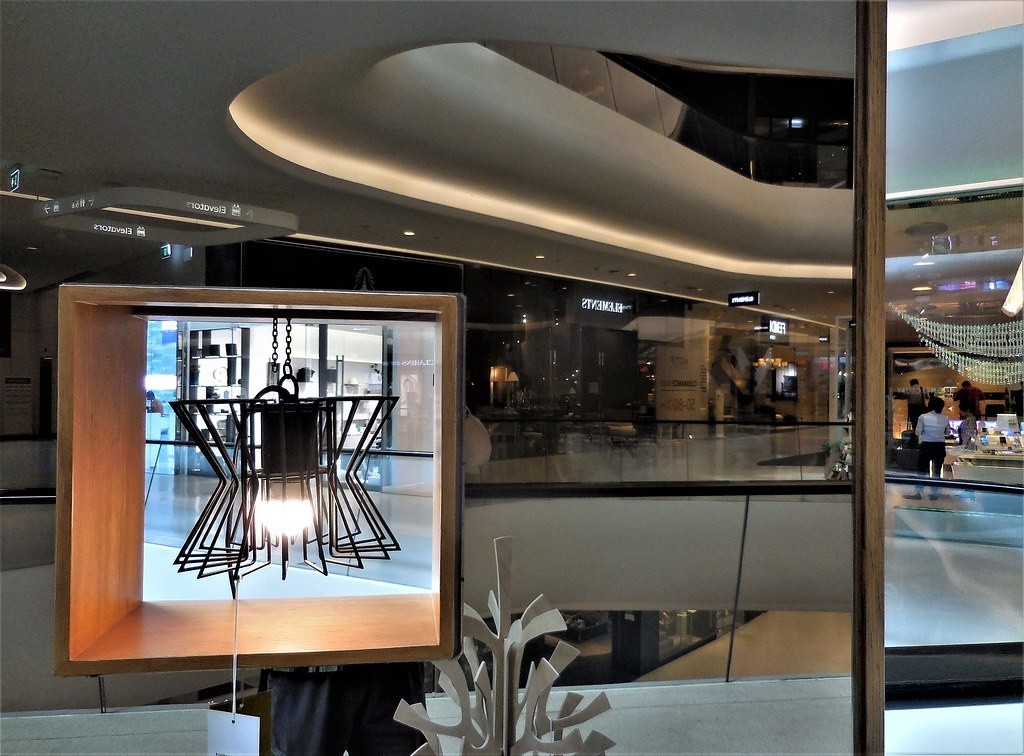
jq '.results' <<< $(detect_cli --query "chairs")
[907,403,927,431]
[959,399,981,420]
[985,404,1005,421]
[901,430,919,449]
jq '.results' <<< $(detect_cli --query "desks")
[930,446,971,478]
[951,453,1024,485]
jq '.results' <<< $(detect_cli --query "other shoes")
[928,494,938,500]
[902,492,922,500]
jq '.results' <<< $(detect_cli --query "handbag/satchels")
[920,387,928,414]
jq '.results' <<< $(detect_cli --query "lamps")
[170,316,401,599]
[507,372,519,407]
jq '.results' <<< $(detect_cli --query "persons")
[953,381,982,452]
[837,366,847,419]
[1015,381,1024,419]
[903,395,951,501]
[904,379,930,431]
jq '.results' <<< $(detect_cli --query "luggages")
[900,418,919,450]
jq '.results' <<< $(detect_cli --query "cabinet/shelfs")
[178,321,250,477]
